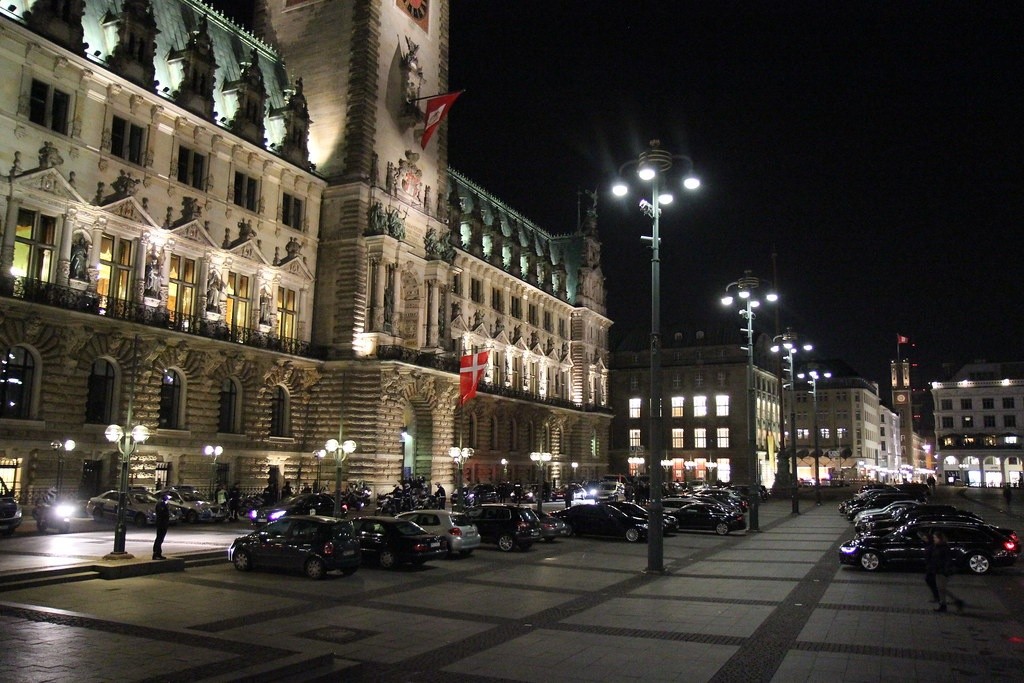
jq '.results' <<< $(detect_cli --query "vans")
[0,476,22,532]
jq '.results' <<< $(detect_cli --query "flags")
[459,350,491,405]
[897,335,909,343]
[420,91,464,150]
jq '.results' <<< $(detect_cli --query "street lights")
[683,459,696,480]
[626,456,645,476]
[797,360,835,508]
[50,439,75,500]
[572,463,578,478]
[612,142,700,574]
[770,328,815,515]
[722,271,778,532]
[661,459,673,482]
[325,438,356,517]
[530,451,551,513]
[706,462,717,482]
[205,445,224,498]
[312,450,328,491]
[448,447,477,512]
[501,458,508,479]
[106,423,149,549]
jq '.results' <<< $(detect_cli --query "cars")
[661,479,731,495]
[86,485,231,527]
[837,482,1022,574]
[450,474,629,506]
[662,484,769,533]
[226,503,678,582]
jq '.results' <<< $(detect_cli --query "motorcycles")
[340,489,439,515]
[32,499,74,534]
[239,492,269,519]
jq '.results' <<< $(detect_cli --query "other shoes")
[933,602,947,612]
[152,555,166,560]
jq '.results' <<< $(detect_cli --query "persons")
[923,532,965,613]
[1003,485,1012,506]
[499,479,573,507]
[153,493,175,559]
[926,475,936,489]
[624,479,677,507]
[215,483,243,524]
[36,487,62,525]
[264,475,446,509]
